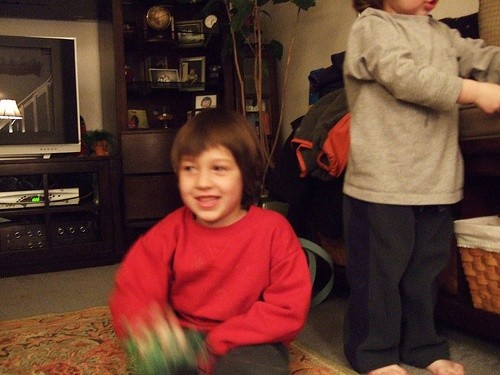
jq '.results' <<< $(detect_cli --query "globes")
[143,4,176,42]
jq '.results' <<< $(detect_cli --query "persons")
[111,110,312,375]
[342,1,500,375]
[197,96,213,114]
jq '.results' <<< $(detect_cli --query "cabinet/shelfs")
[112,1,235,229]
[0,155,125,278]
[235,43,280,160]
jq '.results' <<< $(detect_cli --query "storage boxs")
[454,216,500,313]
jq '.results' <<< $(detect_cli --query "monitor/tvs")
[0,34,82,161]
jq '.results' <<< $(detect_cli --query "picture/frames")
[149,69,178,89]
[175,20,203,46]
[179,56,206,91]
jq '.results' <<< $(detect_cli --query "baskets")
[452,216,500,313]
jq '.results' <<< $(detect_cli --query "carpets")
[0,305,360,375]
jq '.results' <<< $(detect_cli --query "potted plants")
[83,129,113,156]
[225,0,320,238]
